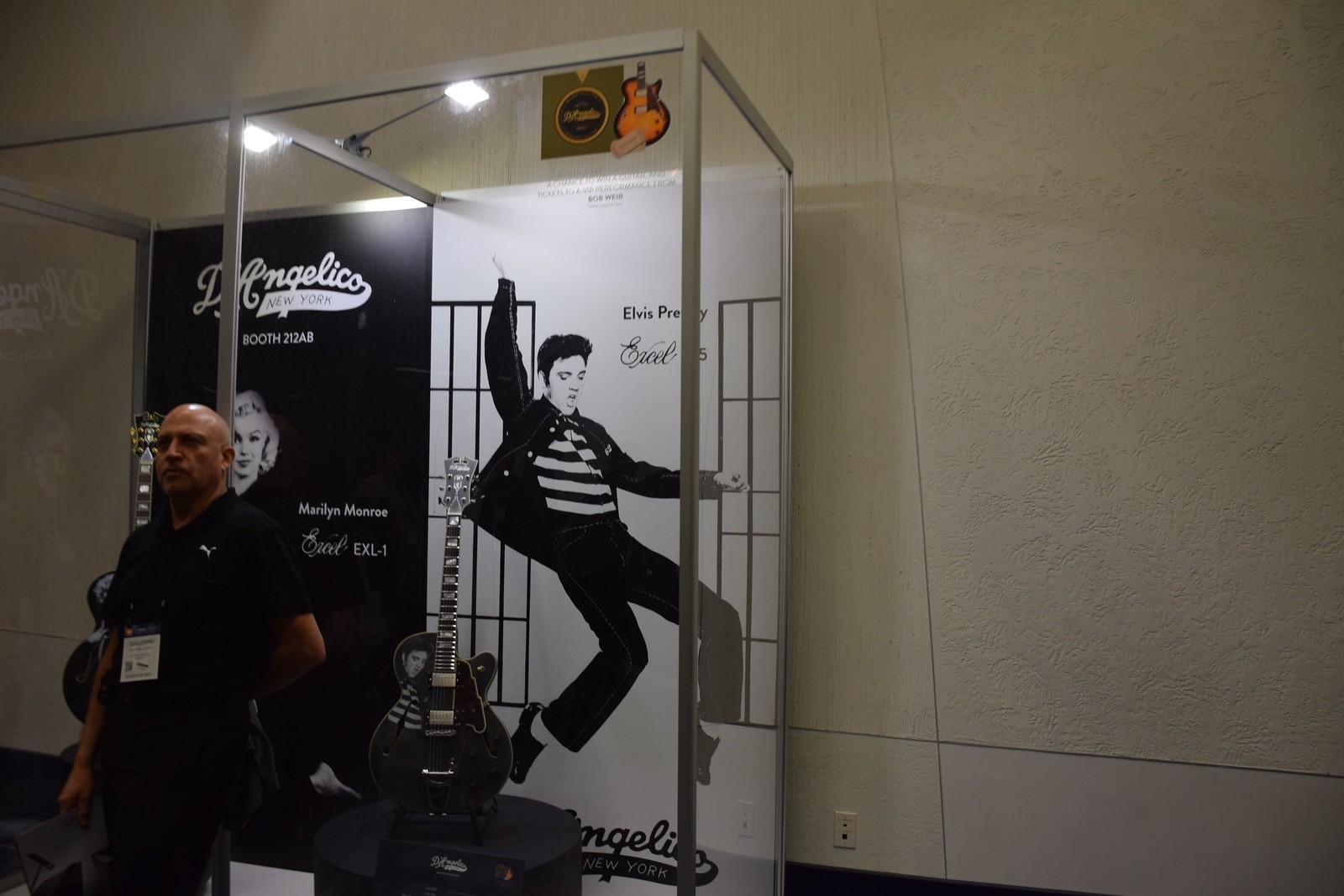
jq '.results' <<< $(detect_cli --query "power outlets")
[735,801,754,838]
[834,809,856,850]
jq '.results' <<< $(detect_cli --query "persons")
[232,391,321,624]
[396,629,436,691]
[52,403,329,895]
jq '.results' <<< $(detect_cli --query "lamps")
[342,76,490,160]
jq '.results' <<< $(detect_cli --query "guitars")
[62,410,165,725]
[369,455,514,814]
